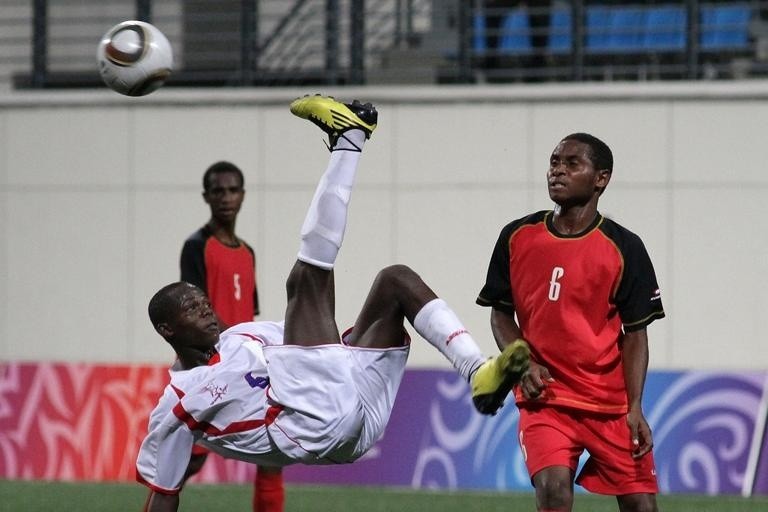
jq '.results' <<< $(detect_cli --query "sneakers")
[470,340,531,416]
[289,94,378,147]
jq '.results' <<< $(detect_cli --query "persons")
[134,93,531,512]
[476,133,665,512]
[145,162,282,512]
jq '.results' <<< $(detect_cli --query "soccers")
[96,21,172,95]
